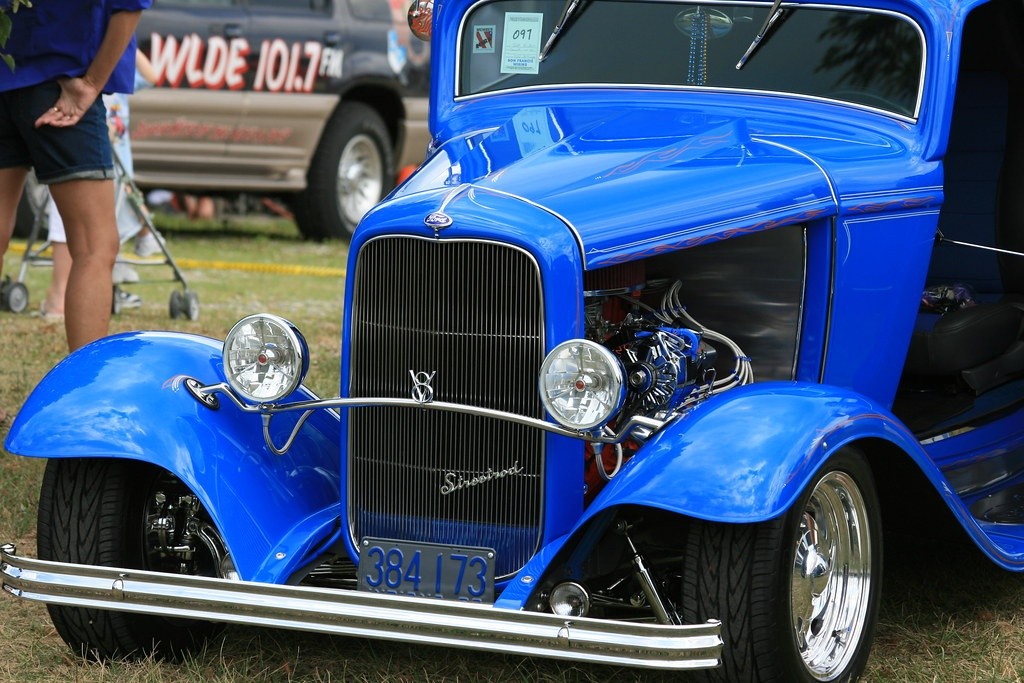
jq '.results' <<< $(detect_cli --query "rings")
[51,107,57,110]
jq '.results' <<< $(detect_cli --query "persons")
[0,0,152,356]
[42,44,168,322]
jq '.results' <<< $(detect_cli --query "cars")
[12,0,405,250]
[1,1,1021,680]
